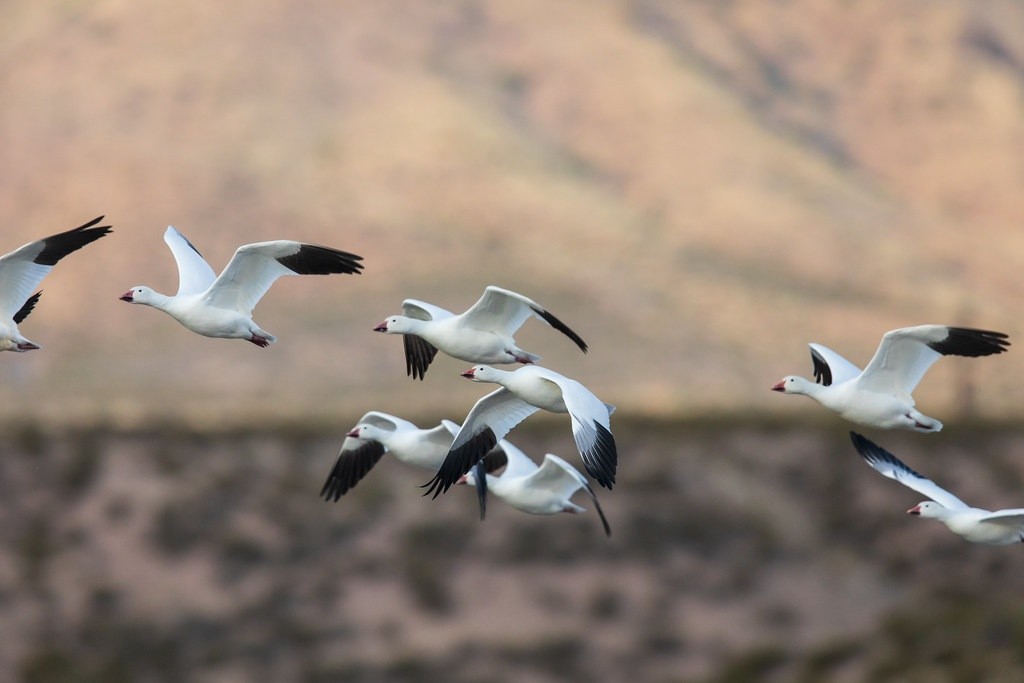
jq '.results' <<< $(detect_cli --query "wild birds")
[849,430,1024,547]
[768,323,1013,434]
[119,224,367,349]
[314,285,619,534]
[1,214,113,353]
